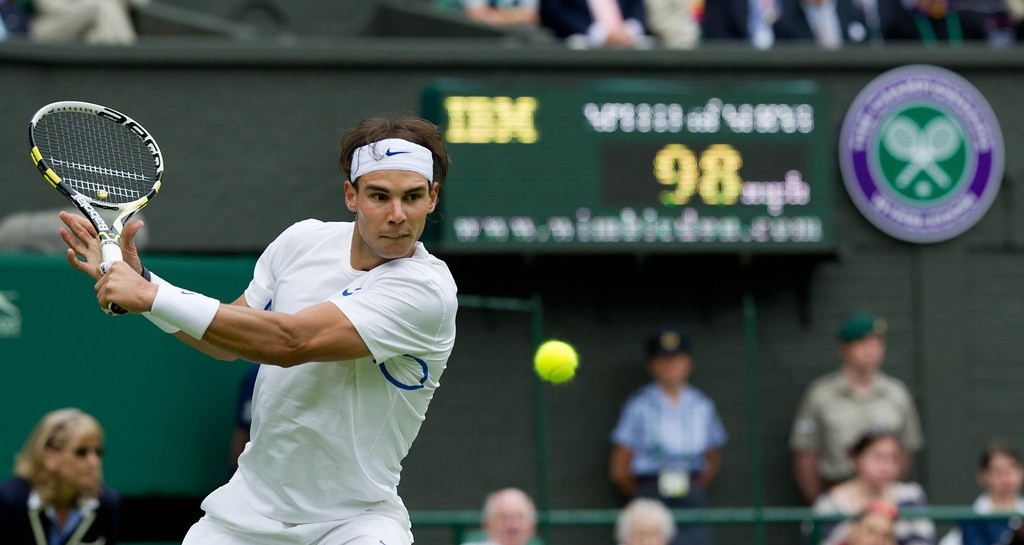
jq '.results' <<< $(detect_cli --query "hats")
[837,316,886,343]
[651,331,688,356]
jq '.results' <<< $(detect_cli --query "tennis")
[534,339,578,384]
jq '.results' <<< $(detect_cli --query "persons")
[819,430,935,545]
[58,116,457,544]
[832,512,893,545]
[790,313,926,502]
[482,489,536,545]
[436,0,1024,47]
[939,445,1024,545]
[617,500,675,545]
[0,409,120,545]
[610,334,726,545]
[0,0,137,45]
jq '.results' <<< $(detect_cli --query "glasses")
[72,447,106,459]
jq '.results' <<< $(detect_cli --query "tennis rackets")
[30,101,165,315]
